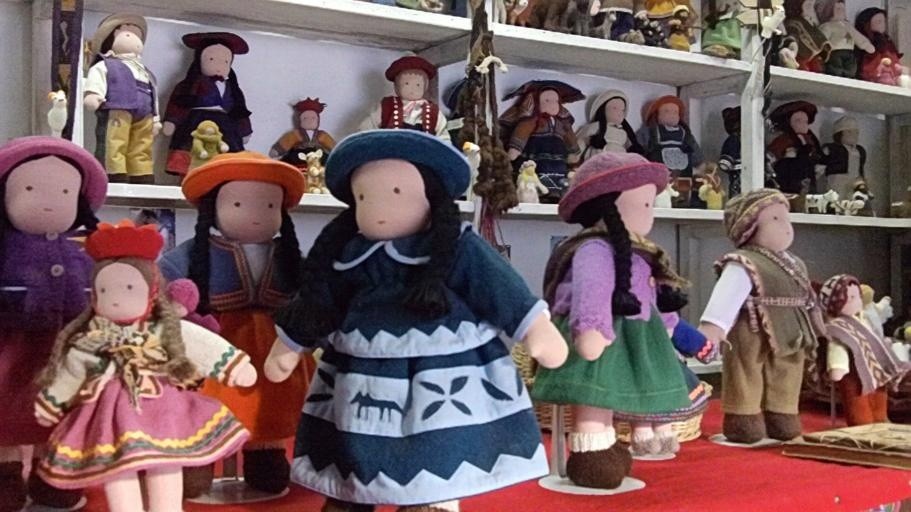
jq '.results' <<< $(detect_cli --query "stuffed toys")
[357,51,480,202]
[35,219,257,512]
[818,274,910,427]
[757,1,910,89]
[498,80,724,210]
[718,100,874,216]
[84,11,161,185]
[364,0,485,19]
[263,130,568,512]
[162,32,253,186]
[156,150,317,498]
[697,187,826,444]
[1,90,109,512]
[269,96,337,174]
[493,1,742,60]
[531,151,716,490]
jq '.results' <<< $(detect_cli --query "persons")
[0,0,911,512]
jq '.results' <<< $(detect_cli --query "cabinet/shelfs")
[672,0,910,356]
[63,0,486,214]
[482,1,759,390]
